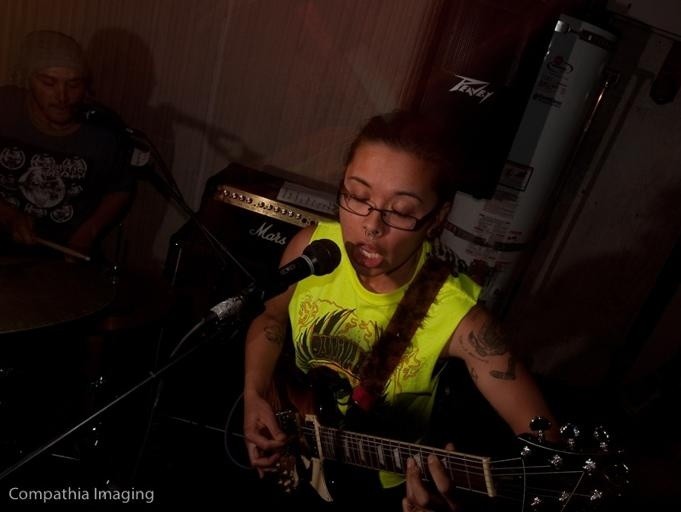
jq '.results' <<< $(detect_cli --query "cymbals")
[0,238,123,337]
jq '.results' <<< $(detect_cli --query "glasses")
[335,191,443,233]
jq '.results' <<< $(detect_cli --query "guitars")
[245,366,633,511]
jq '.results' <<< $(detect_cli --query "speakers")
[188,162,340,329]
[410,0,562,199]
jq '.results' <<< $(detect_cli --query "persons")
[1,29,142,273]
[239,106,574,511]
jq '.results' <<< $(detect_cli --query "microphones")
[70,103,146,140]
[208,239,340,329]
